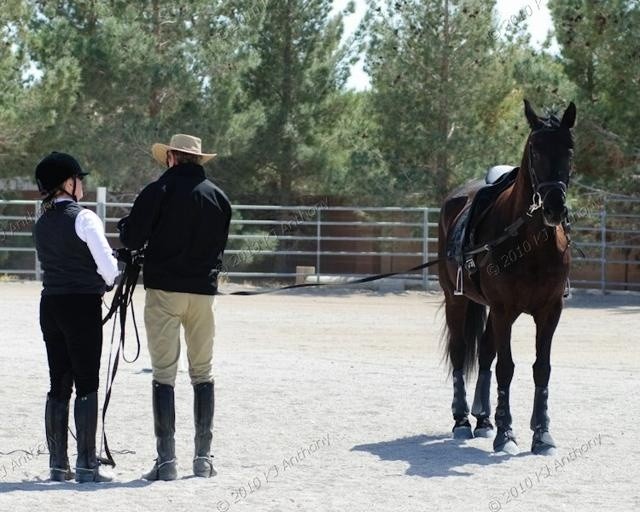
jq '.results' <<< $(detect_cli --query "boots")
[46,401,75,482]
[194,382,217,479]
[141,380,178,481]
[74,391,113,483]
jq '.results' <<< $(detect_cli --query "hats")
[152,133,217,167]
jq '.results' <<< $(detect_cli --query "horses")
[436,95,578,457]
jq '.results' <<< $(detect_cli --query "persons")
[116,133,234,482]
[31,150,124,484]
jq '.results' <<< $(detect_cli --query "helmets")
[35,151,89,195]
